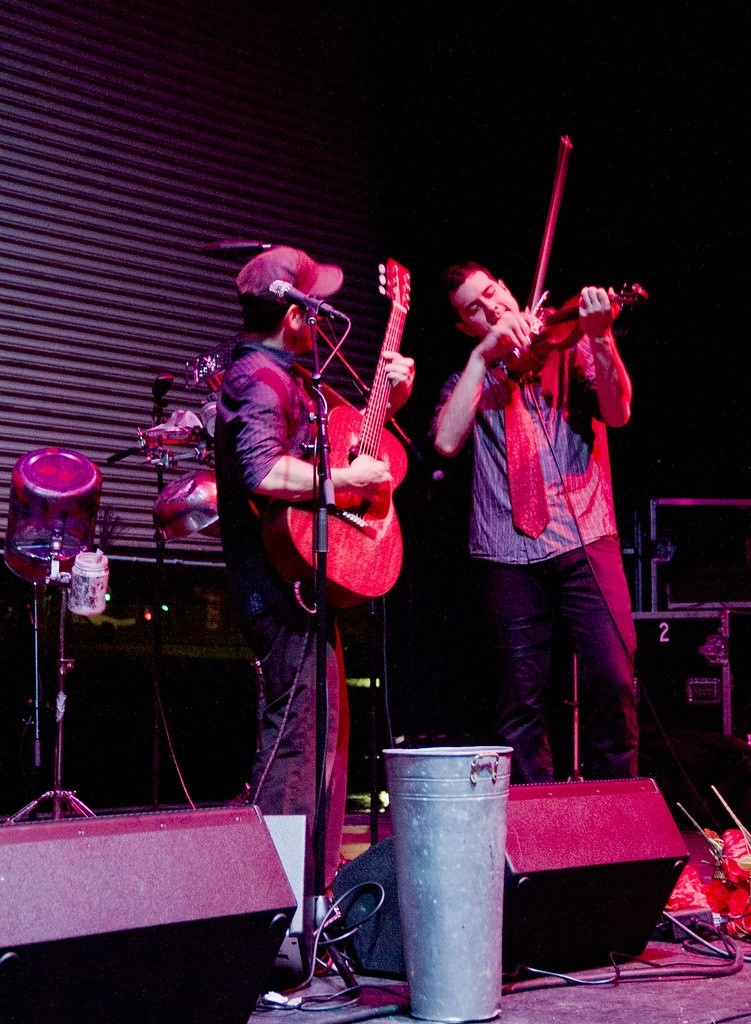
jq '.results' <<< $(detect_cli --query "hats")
[234,246,345,303]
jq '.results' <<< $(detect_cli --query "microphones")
[268,280,344,319]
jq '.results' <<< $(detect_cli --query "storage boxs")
[571,609,751,827]
[648,497,751,609]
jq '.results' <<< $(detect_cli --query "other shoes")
[260,975,365,1011]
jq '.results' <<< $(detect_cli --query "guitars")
[261,258,415,613]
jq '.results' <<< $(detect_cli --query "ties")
[487,363,551,540]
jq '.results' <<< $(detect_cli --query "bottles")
[68,553,109,616]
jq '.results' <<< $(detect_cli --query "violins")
[510,279,648,370]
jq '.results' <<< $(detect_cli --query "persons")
[431,263,637,785]
[214,248,416,902]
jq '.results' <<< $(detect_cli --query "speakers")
[332,777,690,982]
[0,805,298,1024]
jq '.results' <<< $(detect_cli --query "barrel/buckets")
[382,747,514,1022]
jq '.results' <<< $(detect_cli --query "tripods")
[3,572,99,824]
